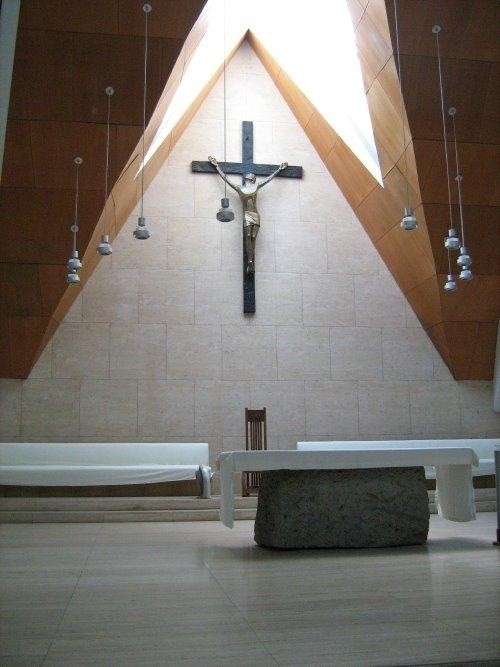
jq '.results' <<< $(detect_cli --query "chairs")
[240,406,268,497]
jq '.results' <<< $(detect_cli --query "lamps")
[433,24,459,255]
[447,106,474,272]
[68,222,84,286]
[215,11,240,228]
[69,155,82,275]
[439,251,454,297]
[389,3,421,231]
[455,262,477,290]
[97,85,115,257]
[134,3,153,246]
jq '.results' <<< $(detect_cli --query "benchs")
[295,438,499,486]
[1,438,215,500]
[218,445,481,527]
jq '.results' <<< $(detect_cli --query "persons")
[208,154,290,273]
